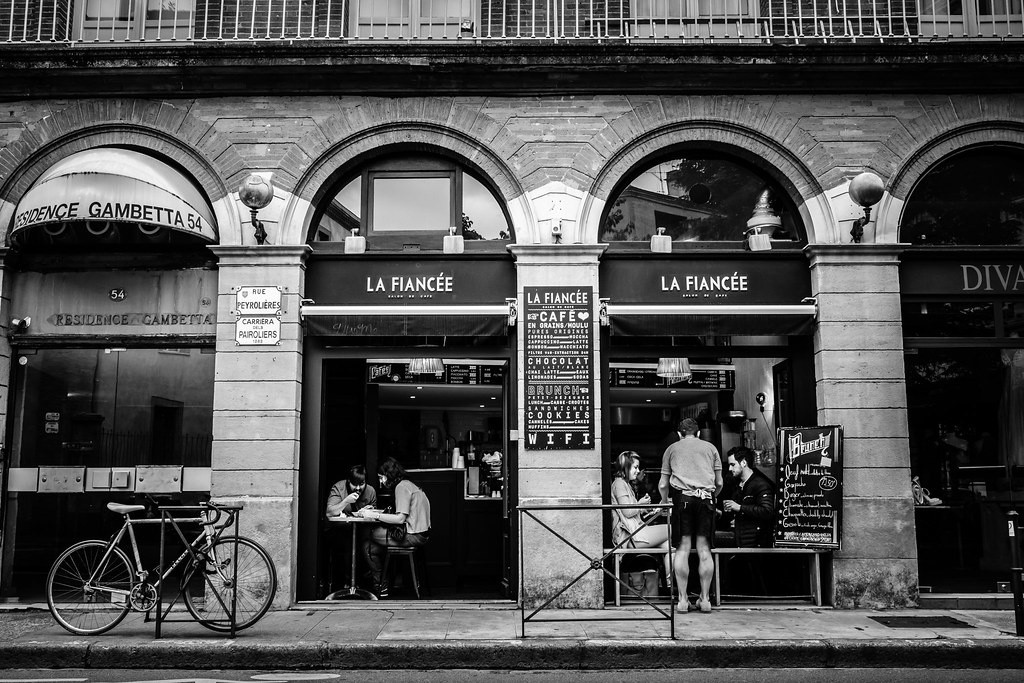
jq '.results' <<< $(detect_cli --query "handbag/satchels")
[386,523,407,542]
[621,570,659,598]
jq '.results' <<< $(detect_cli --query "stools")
[380,546,422,600]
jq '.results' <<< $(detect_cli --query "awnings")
[9,148,216,247]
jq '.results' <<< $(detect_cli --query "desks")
[914,504,966,593]
[326,516,382,602]
[712,531,735,606]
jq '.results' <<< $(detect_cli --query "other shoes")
[695,594,713,613]
[677,599,689,612]
[374,589,389,598]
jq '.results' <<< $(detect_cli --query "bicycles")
[45,501,277,636]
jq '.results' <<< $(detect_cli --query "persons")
[655,418,723,612]
[611,451,696,595]
[326,460,431,596]
[710,446,777,593]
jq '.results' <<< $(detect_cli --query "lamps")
[444,227,464,253]
[849,172,884,243]
[238,176,275,245]
[407,334,447,374]
[345,227,367,254]
[651,227,673,253]
[748,226,772,252]
[657,336,692,376]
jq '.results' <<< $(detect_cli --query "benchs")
[603,547,833,607]
